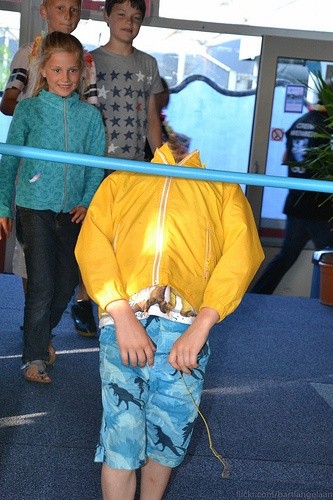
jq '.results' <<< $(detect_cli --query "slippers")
[22,360,52,383]
[46,344,55,366]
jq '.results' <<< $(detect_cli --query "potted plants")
[297,70,333,306]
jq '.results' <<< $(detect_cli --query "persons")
[0,31,106,382]
[155,76,178,148]
[1,1,99,112]
[70,0,165,337]
[251,82,333,298]
[74,139,266,499]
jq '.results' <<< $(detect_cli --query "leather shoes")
[70,300,98,336]
